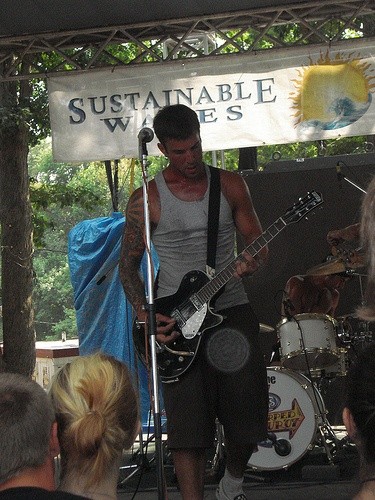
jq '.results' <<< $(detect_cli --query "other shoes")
[215,478,248,500]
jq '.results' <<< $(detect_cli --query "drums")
[222,365,326,472]
[277,312,339,370]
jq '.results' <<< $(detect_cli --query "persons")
[1,371,94,500]
[118,103,269,500]
[48,351,140,500]
[342,340,375,500]
[269,251,353,435]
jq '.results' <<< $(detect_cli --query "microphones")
[283,290,295,312]
[138,128,154,143]
[275,439,291,456]
[336,161,343,189]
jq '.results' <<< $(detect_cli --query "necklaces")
[360,477,375,487]
[65,486,115,500]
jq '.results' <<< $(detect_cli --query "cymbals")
[308,253,369,276]
[336,312,359,319]
[259,321,275,333]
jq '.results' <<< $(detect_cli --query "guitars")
[132,187,324,379]
